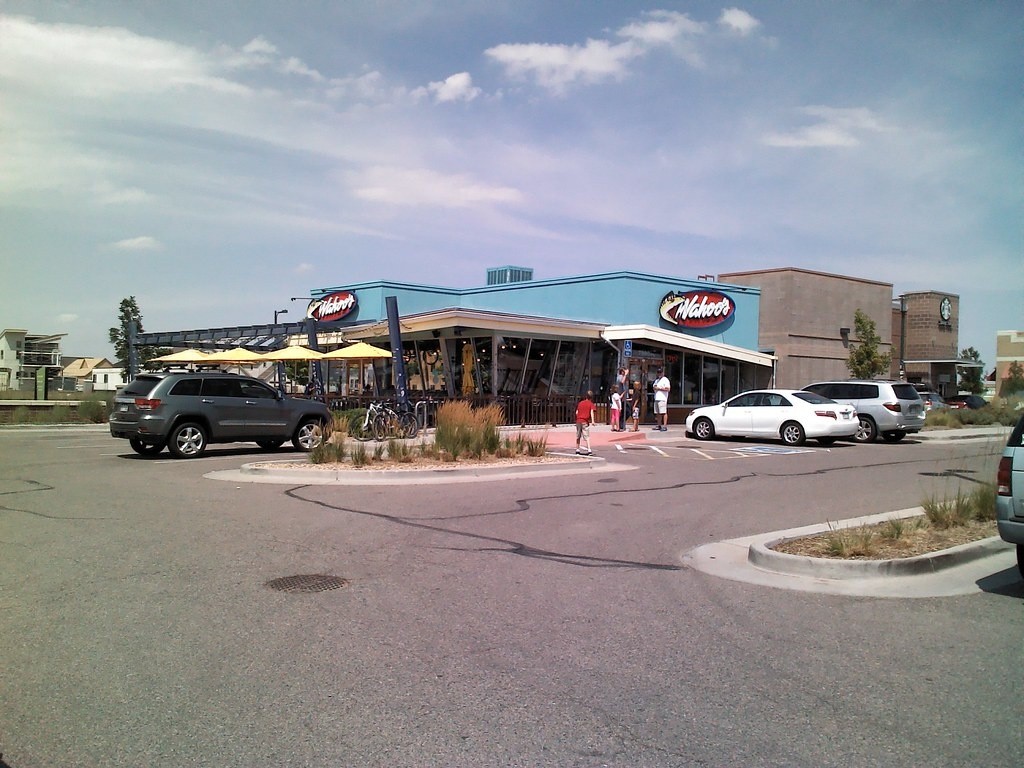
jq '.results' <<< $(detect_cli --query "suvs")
[798,378,927,444]
[109,363,332,460]
[996,414,1024,580]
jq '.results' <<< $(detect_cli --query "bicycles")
[349,399,420,442]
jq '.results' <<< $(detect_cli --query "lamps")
[433,329,441,338]
[454,330,463,337]
[841,326,851,334]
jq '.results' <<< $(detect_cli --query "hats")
[657,368,663,373]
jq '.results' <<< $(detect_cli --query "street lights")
[275,309,289,325]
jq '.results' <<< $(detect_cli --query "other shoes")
[660,427,667,431]
[576,452,580,455]
[588,452,593,456]
[652,425,661,430]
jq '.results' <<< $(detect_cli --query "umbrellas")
[197,348,265,375]
[148,348,210,369]
[260,346,326,382]
[318,342,406,396]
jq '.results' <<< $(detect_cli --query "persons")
[651,368,671,431]
[616,366,633,432]
[575,390,598,454]
[609,384,626,432]
[627,382,641,432]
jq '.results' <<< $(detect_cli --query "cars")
[945,394,987,410]
[686,389,860,445]
[916,391,946,413]
